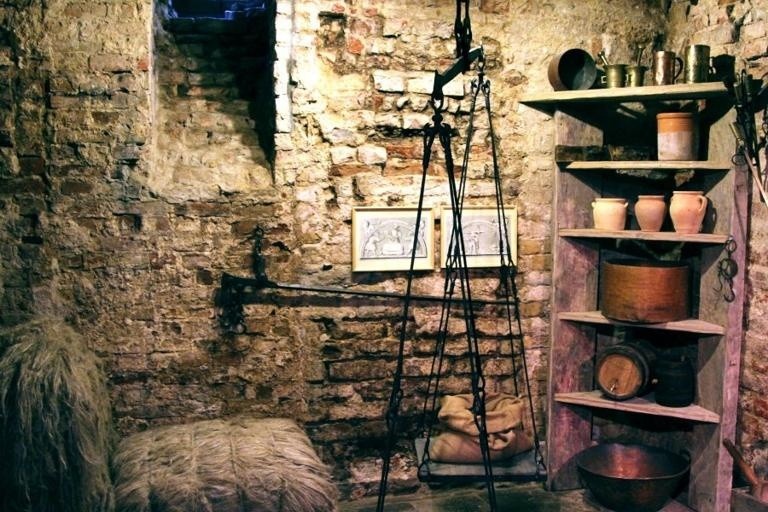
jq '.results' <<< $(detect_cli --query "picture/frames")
[440,205,517,270]
[352,207,436,273]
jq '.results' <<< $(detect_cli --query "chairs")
[0,314,343,511]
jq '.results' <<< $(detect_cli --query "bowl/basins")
[547,48,598,91]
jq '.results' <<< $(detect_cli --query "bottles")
[650,354,695,407]
[633,193,667,231]
[592,197,628,231]
[670,189,708,234]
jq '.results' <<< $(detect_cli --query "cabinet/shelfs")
[518,82,750,512]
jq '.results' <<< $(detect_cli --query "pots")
[573,440,693,509]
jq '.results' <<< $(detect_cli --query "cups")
[656,113,699,164]
[600,44,714,88]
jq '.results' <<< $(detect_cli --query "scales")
[377,0,561,512]
[218,228,517,336]
[729,122,768,209]
